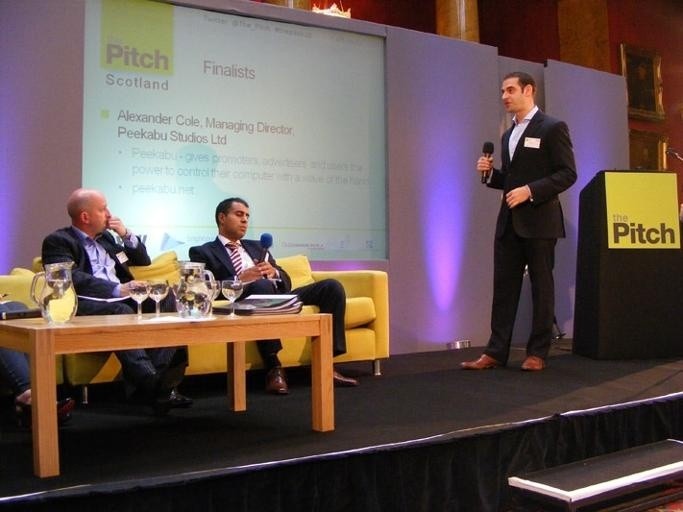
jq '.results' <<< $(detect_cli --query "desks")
[1,313,336,479]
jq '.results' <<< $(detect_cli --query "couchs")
[0,255,389,405]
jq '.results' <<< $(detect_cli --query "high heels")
[17,393,76,432]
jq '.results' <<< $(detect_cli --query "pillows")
[275,253,314,291]
[129,250,183,290]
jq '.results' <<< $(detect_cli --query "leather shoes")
[521,356,546,370]
[461,353,508,370]
[265,367,288,394]
[140,392,171,416]
[166,390,196,407]
[332,370,360,388]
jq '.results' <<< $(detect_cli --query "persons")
[42,190,193,405]
[458,72,577,372]
[0,299,74,432]
[189,197,359,394]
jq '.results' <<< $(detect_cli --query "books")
[207,291,303,315]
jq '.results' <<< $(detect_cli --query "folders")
[211,294,303,314]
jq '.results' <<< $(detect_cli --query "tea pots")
[172,261,219,321]
[28,261,80,326]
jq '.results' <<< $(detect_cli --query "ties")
[228,242,242,277]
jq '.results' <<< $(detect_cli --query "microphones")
[259,233,273,263]
[481,142,494,183]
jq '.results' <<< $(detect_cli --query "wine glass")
[126,278,244,320]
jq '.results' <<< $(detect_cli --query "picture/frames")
[619,42,672,173]
